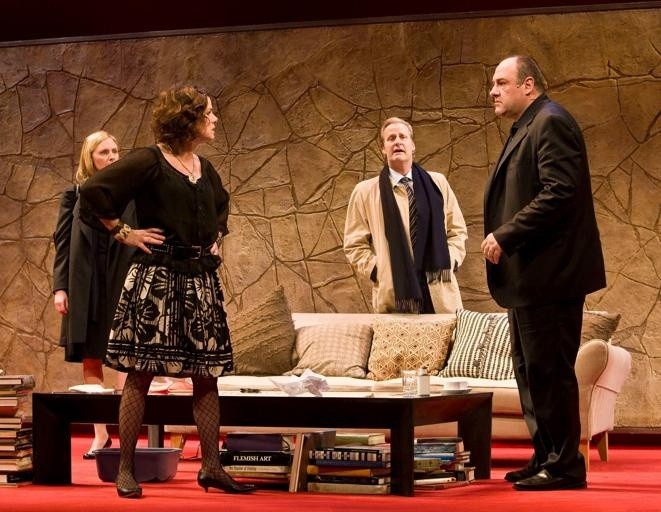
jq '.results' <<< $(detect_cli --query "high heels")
[114,473,143,497]
[196,465,256,494]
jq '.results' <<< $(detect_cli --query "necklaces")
[168,144,195,181]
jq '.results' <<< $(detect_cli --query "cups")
[401,369,417,396]
[444,381,466,389]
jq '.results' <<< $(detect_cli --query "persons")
[342,117,468,314]
[52,130,119,460]
[480,55,607,490]
[73,83,256,496]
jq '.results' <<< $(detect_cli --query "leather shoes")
[82,433,113,460]
[503,451,588,491]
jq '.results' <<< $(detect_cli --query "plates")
[439,389,472,395]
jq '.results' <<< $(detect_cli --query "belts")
[146,241,213,261]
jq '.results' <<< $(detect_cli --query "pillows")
[576,305,620,347]
[437,307,515,378]
[228,285,298,378]
[281,320,375,378]
[367,316,457,377]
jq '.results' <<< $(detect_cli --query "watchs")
[118,224,132,243]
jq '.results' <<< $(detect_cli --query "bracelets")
[215,236,222,249]
[111,223,123,236]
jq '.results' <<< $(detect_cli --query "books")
[0,375,35,488]
[219,433,476,495]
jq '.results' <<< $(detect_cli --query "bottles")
[418,366,430,399]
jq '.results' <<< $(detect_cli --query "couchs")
[290,305,632,474]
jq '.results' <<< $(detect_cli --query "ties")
[400,173,421,261]
